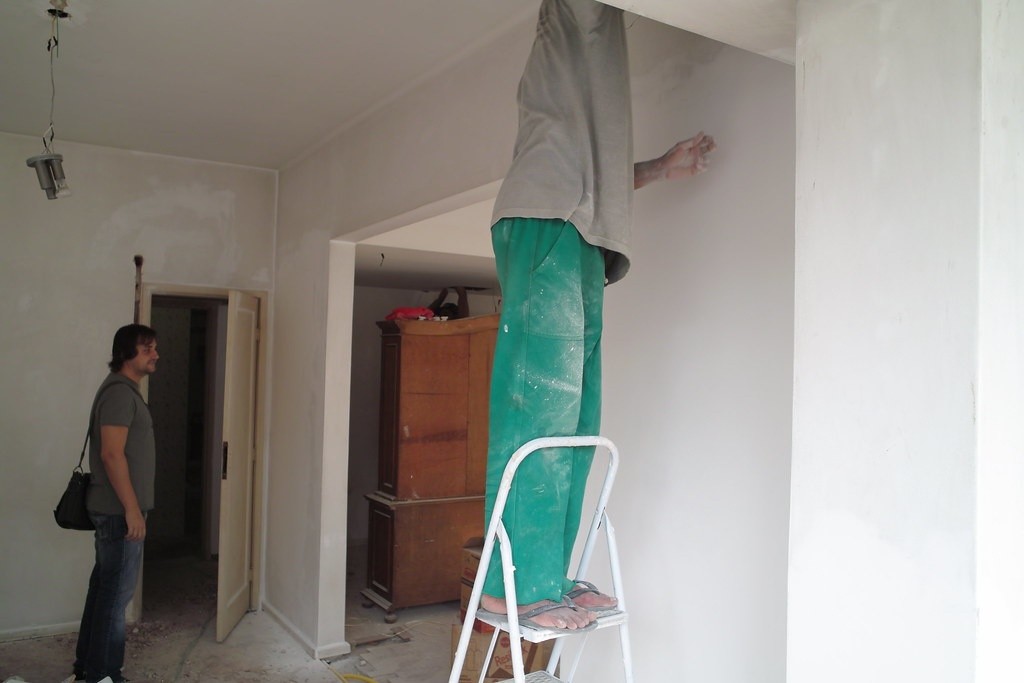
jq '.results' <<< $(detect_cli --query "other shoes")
[72,670,129,683]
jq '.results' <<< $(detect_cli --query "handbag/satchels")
[55,472,98,530]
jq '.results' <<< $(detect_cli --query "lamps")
[26,153,73,201]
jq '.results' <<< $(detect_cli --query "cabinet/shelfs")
[360,306,502,626]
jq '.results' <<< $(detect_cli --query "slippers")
[565,580,622,618]
[476,595,598,634]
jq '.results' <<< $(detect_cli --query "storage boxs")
[451,535,562,683]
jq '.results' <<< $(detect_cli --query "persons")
[73,324,160,683]
[474,0,717,635]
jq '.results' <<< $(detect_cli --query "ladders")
[447,435,633,683]
